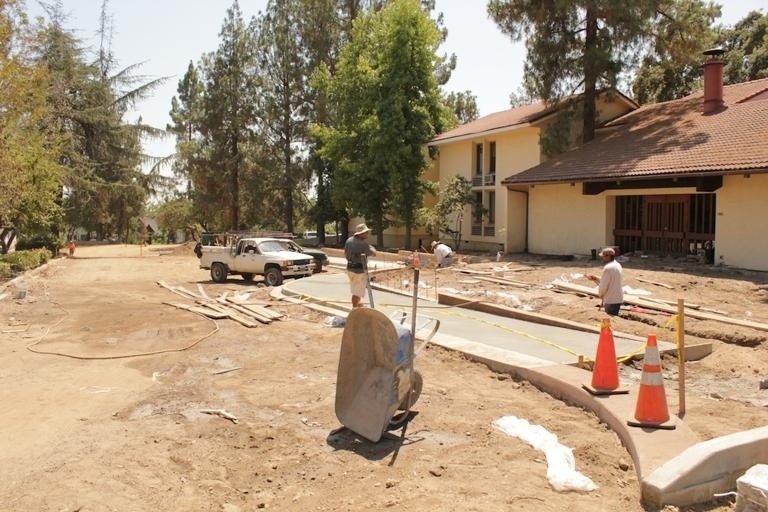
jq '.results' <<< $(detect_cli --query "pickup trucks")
[196,236,316,286]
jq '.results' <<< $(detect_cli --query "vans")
[303,231,326,239]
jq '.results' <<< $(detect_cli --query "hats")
[431,241,440,250]
[353,224,372,235]
[599,248,615,258]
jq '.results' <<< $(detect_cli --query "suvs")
[236,238,329,274]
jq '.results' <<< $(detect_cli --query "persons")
[586,247,622,316]
[342,224,375,308]
[66,241,75,256]
[429,240,452,268]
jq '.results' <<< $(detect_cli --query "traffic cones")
[623,332,675,431]
[580,318,629,395]
[412,250,420,266]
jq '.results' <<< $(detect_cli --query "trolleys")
[332,253,442,444]
